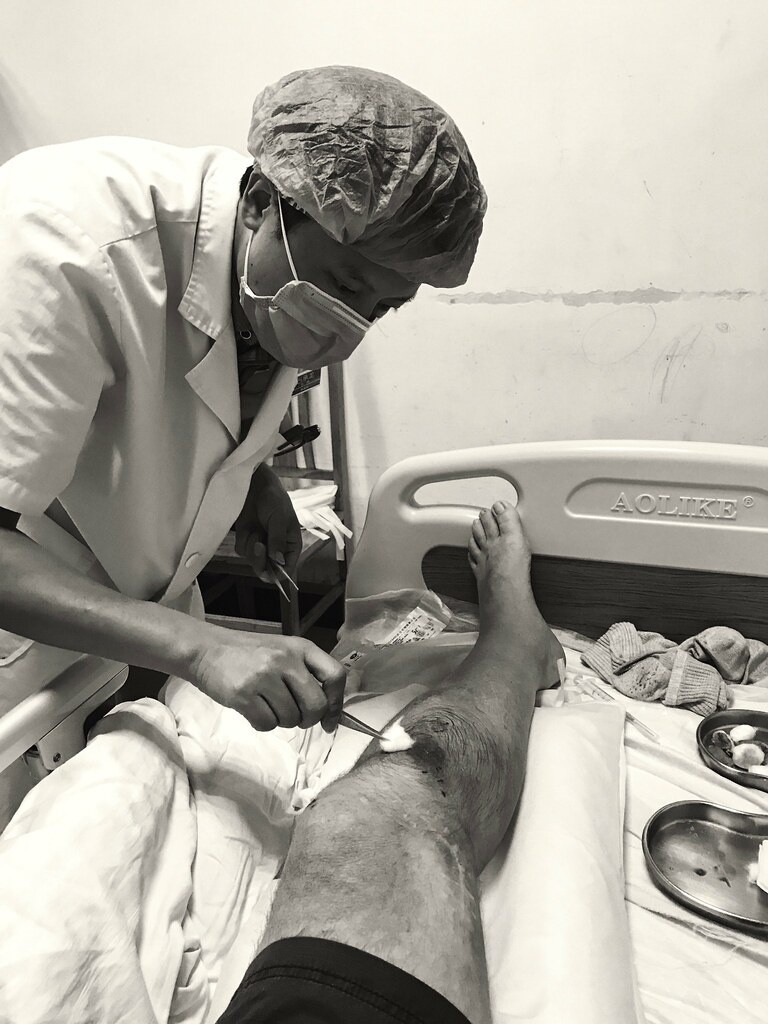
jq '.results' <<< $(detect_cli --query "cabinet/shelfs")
[199,346,356,639]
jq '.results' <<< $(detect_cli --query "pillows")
[310,702,639,1023]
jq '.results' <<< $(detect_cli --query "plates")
[641,800,768,939]
[697,710,768,790]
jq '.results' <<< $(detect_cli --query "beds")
[0,437,768,1024]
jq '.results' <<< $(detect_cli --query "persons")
[216,500,566,1024]
[0,65,488,836]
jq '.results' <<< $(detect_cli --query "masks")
[239,191,380,371]
